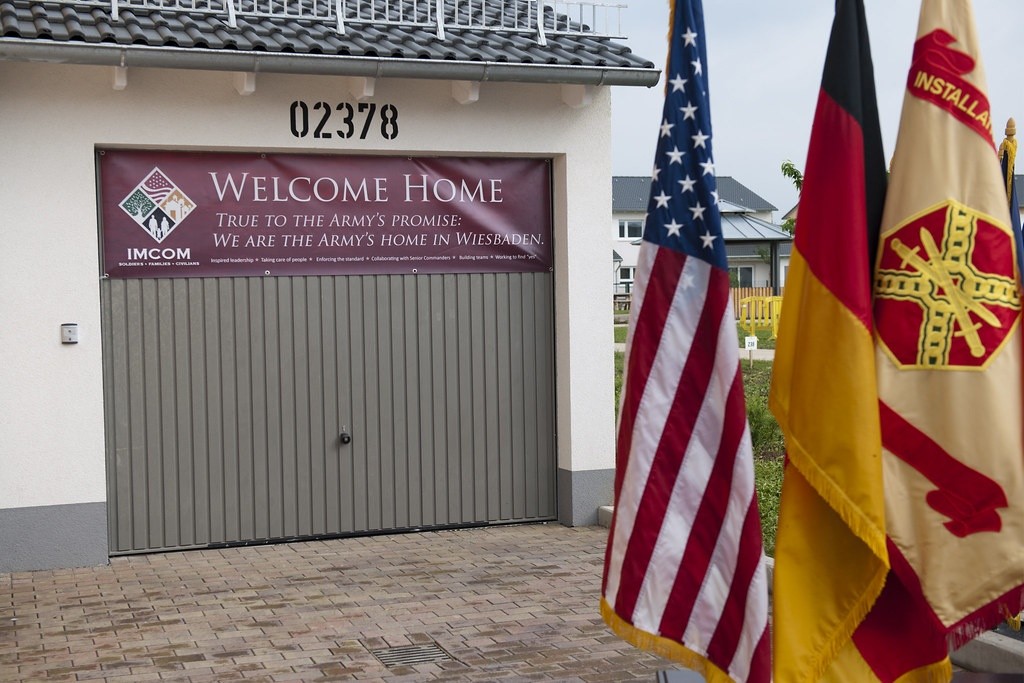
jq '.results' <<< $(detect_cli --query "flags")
[596,0,1024,682]
